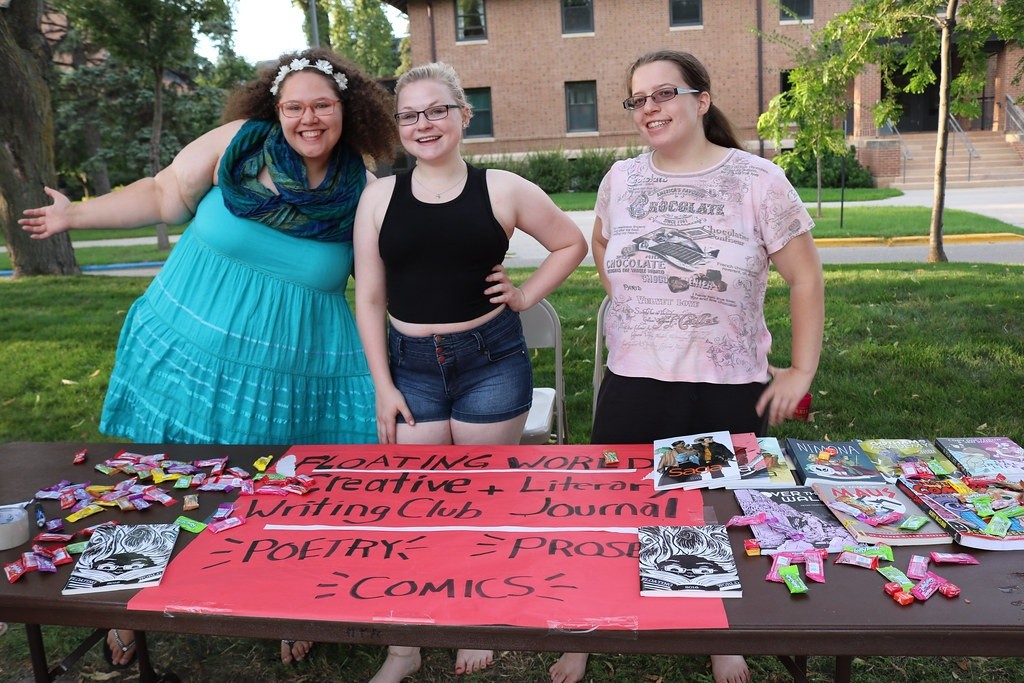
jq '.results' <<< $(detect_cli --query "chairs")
[592,294,610,432]
[518,299,570,445]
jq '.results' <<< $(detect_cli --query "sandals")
[280,639,313,666]
[104,629,138,667]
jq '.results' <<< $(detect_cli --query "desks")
[0,442,1024,683]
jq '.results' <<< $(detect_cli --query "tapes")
[0,507,30,551]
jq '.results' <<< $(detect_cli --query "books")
[637,525,744,600]
[60,524,180,594]
[653,430,1023,556]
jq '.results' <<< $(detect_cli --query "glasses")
[276,97,343,118]
[394,104,460,126]
[622,87,700,110]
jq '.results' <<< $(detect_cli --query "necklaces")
[413,171,467,199]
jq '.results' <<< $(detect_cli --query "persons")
[16,47,396,671]
[352,63,591,683]
[546,46,828,683]
